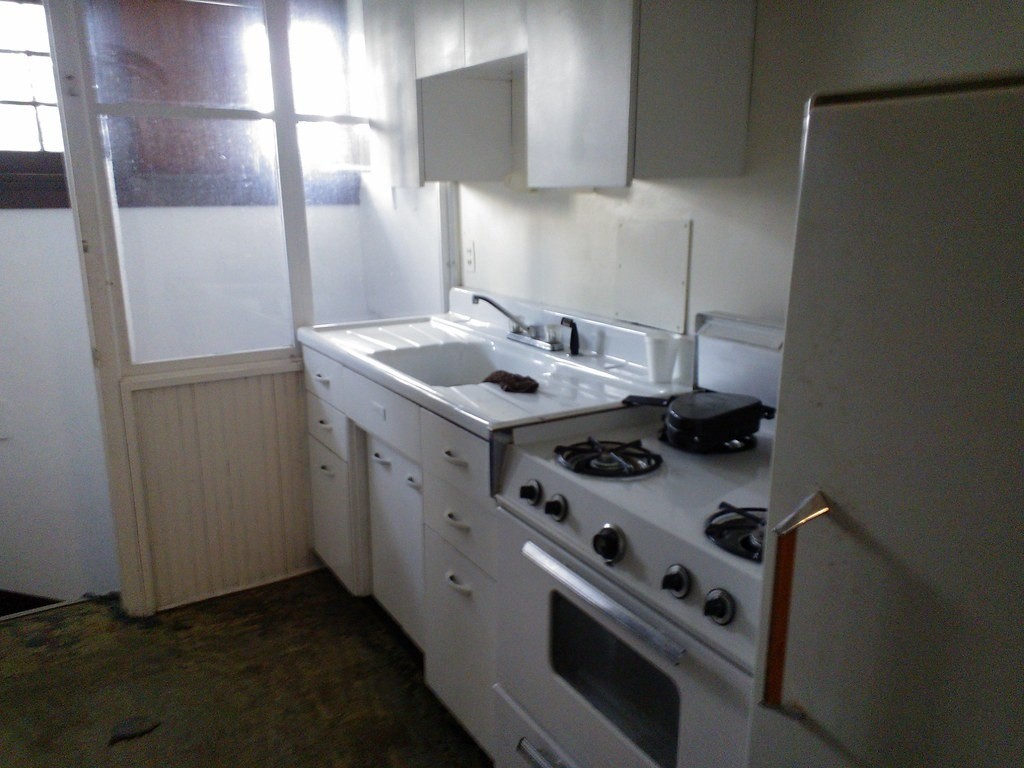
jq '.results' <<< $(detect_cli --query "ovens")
[495,506,756,768]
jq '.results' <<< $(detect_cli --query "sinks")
[370,342,556,387]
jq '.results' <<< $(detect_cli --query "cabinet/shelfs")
[301,345,503,757]
[362,0,757,186]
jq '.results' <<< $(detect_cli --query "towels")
[481,370,540,394]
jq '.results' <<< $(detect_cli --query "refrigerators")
[748,78,1024,768]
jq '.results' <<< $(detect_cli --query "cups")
[644,333,682,385]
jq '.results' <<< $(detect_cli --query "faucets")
[473,295,545,339]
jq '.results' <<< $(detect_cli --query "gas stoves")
[494,312,791,676]
